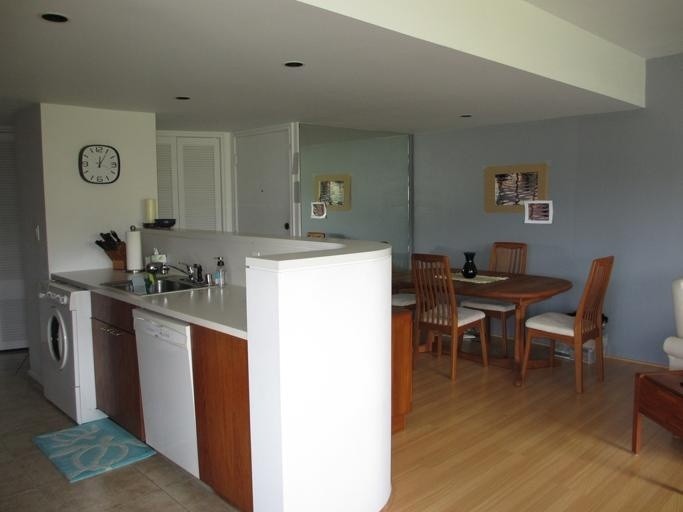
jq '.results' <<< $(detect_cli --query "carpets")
[29,416,157,483]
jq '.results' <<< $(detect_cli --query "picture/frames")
[310,173,353,221]
[485,162,554,225]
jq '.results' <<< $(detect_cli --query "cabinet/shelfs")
[189,323,256,511]
[91,290,145,445]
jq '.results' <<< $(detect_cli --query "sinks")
[96,274,216,298]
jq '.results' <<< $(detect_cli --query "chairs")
[389,241,614,394]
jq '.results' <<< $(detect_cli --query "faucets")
[160,263,203,283]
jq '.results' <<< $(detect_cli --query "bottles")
[142,198,156,227]
[460,251,479,278]
[213,256,227,287]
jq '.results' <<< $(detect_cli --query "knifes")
[94,231,120,251]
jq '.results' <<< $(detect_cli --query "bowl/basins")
[156,218,176,228]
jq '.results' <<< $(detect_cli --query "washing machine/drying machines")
[26,279,111,425]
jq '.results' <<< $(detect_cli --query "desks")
[632,370,683,455]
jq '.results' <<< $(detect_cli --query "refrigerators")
[129,308,200,483]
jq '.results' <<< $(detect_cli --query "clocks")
[79,144,121,184]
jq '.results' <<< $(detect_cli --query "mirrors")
[297,122,414,273]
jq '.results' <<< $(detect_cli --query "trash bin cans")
[554,312,608,364]
